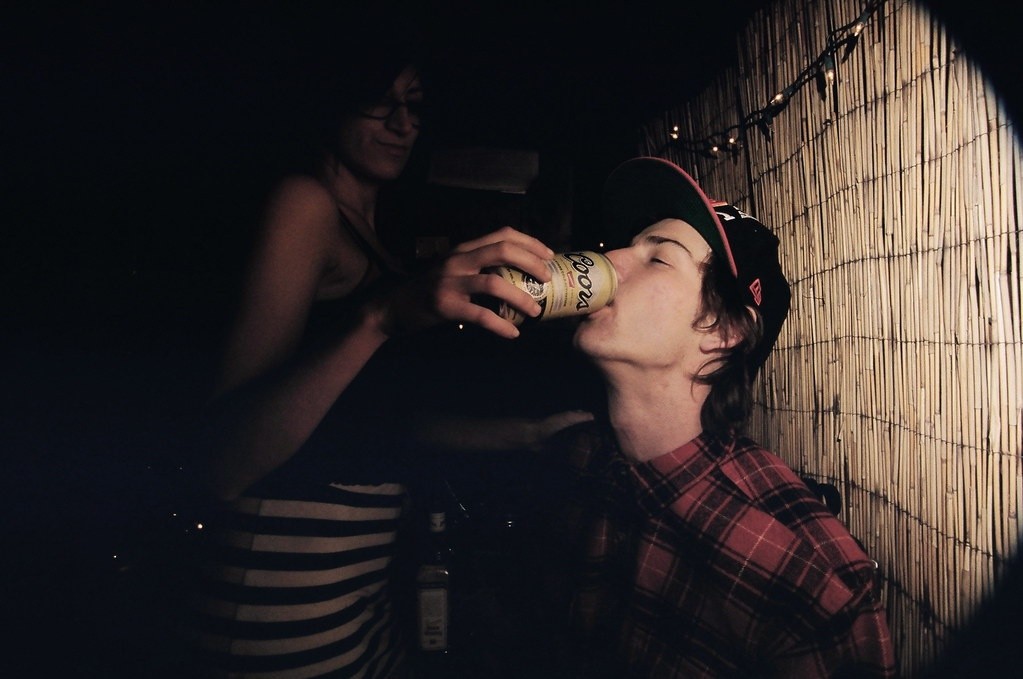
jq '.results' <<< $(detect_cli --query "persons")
[198,64,593,679]
[525,156,898,679]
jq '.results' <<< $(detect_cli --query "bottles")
[417,508,458,659]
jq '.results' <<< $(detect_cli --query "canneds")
[482,251,617,324]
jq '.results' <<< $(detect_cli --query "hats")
[600,156,791,368]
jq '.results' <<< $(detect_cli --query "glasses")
[340,92,432,132]
